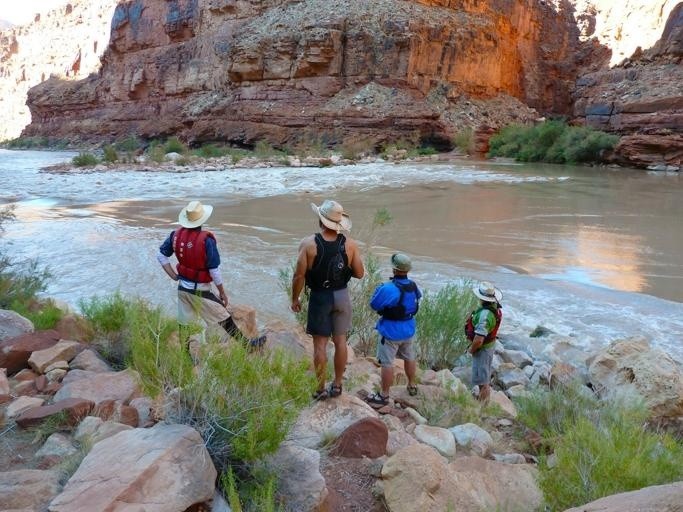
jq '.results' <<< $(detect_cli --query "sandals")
[329,380,342,397]
[406,383,418,396]
[311,389,327,400]
[365,391,390,406]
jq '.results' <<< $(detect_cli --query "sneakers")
[181,358,199,371]
[244,335,267,352]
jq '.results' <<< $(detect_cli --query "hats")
[310,199,352,231]
[177,199,213,230]
[389,252,413,273]
[471,280,503,304]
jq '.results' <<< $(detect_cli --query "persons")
[367,254,421,406]
[465,282,502,404]
[157,202,268,365]
[292,199,364,399]
